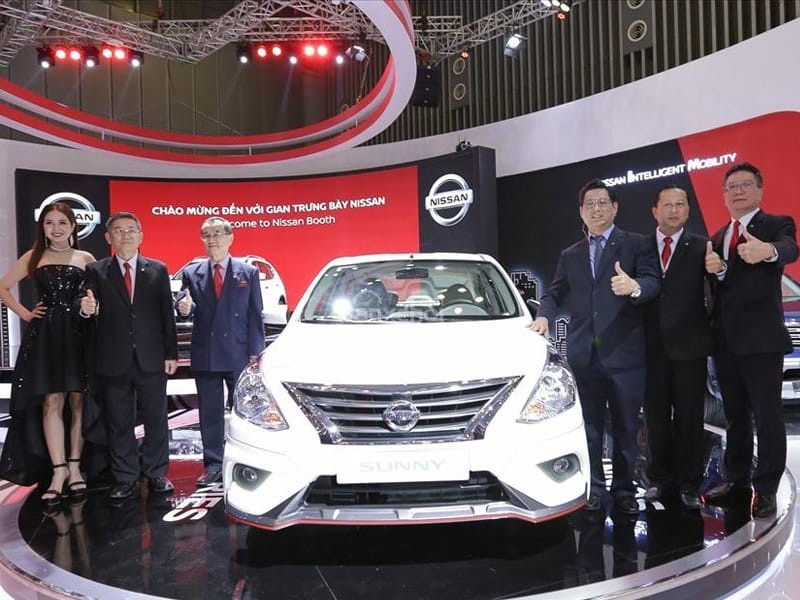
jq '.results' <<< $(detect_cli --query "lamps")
[541,0,572,12]
[503,28,529,59]
[128,44,144,67]
[236,31,367,65]
[82,46,99,67]
[35,43,56,69]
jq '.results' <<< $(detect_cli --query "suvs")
[170,254,288,335]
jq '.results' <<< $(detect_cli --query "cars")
[222,252,591,533]
[704,271,800,425]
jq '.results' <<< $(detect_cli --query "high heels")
[67,458,87,497]
[42,462,69,505]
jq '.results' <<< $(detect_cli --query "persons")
[72,211,180,498]
[42,498,92,571]
[703,162,800,516]
[174,218,267,487]
[643,182,728,512]
[0,203,99,505]
[524,179,662,515]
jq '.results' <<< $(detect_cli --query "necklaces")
[50,244,70,252]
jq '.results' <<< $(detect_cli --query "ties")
[661,236,673,269]
[592,235,604,278]
[123,262,132,303]
[213,263,223,300]
[728,220,741,260]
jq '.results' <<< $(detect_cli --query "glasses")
[724,183,758,193]
[199,231,230,242]
[584,199,612,208]
[112,227,140,236]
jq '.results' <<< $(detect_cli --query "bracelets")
[630,279,642,298]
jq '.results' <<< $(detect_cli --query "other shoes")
[705,481,752,506]
[646,486,663,504]
[751,488,777,517]
[583,494,601,510]
[680,492,702,509]
[614,495,640,514]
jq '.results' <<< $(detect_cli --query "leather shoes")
[147,475,174,493]
[195,471,222,486]
[109,480,139,497]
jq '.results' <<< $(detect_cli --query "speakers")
[619,0,655,56]
[410,69,442,107]
[448,49,472,109]
